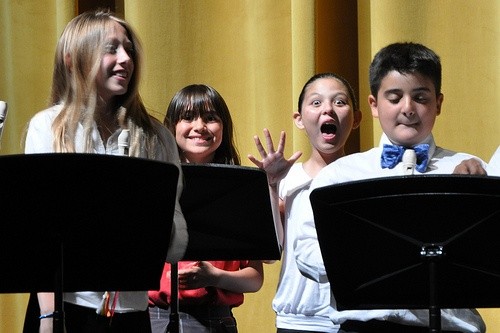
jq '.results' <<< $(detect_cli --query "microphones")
[0,101,8,135]
[402,149,417,175]
[118,129,131,155]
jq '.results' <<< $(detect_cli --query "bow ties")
[380,143,430,173]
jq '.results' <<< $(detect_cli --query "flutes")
[402,149,417,175]
[96,129,132,317]
[0,100,8,136]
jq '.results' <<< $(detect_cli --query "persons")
[148,84,264,333]
[22,10,188,333]
[292,42,490,333]
[248,71,363,333]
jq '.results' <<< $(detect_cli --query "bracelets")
[38,311,54,319]
